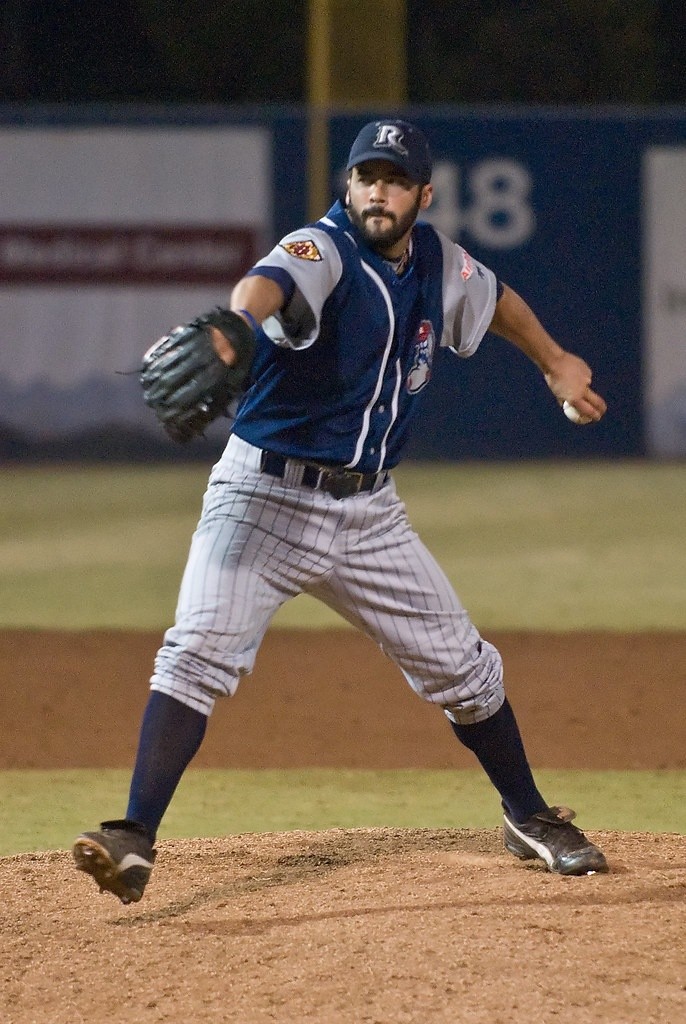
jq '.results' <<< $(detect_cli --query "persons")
[74,117,606,906]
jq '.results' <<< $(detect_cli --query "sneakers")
[73,819,157,904]
[500,798,607,875]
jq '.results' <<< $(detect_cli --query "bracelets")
[240,311,259,332]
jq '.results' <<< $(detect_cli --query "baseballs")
[562,398,595,427]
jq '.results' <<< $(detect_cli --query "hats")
[347,119,431,184]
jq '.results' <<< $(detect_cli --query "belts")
[261,448,389,500]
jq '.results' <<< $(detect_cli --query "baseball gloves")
[136,308,259,446]
